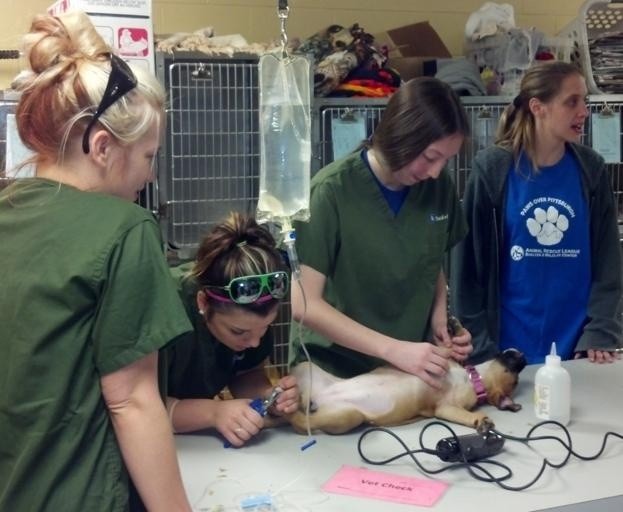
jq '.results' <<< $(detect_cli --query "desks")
[158,350,623,512]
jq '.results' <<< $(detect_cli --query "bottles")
[533,341,572,426]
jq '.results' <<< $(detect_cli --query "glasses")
[82,52,137,155]
[203,271,290,306]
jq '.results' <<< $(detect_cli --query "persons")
[290,76,473,389]
[158,211,300,449]
[449,61,623,364]
[0,12,196,512]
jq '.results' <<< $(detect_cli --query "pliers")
[223,385,284,448]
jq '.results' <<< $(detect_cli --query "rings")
[233,427,242,434]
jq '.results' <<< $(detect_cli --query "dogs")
[260,315,528,439]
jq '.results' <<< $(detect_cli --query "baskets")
[554,1,622,95]
[466,39,573,97]
